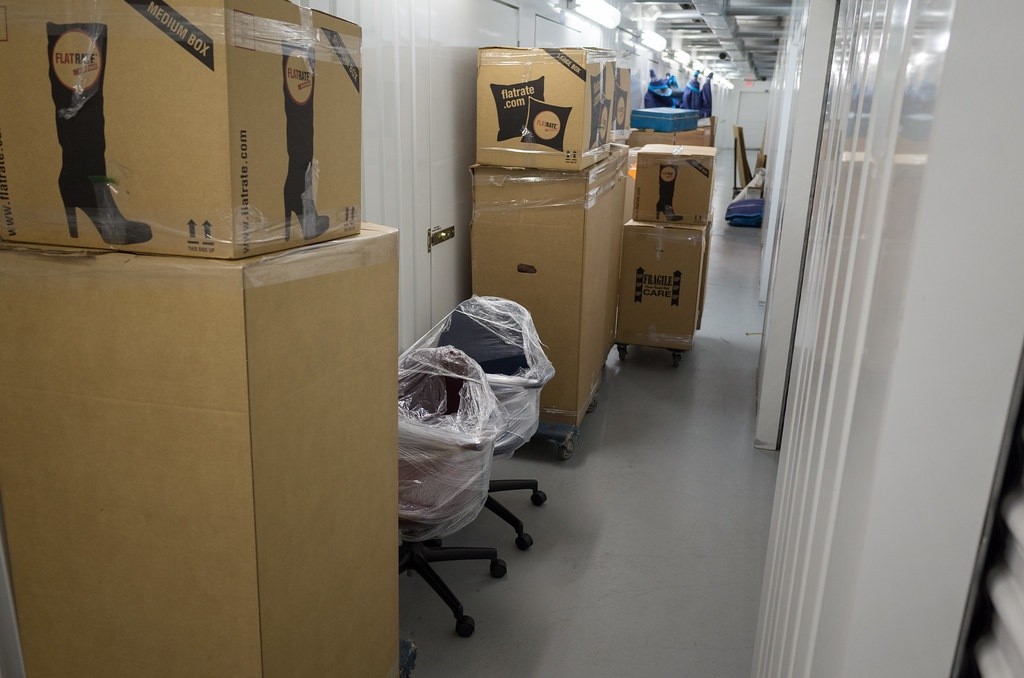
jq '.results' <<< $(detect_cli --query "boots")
[45,19,157,249]
[653,164,684,222]
[280,41,330,241]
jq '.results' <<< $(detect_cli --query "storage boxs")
[0,223,399,678]
[1,0,363,261]
[470,47,717,440]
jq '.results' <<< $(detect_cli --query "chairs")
[438,297,547,552]
[398,343,506,638]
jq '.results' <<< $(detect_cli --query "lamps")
[567,0,622,29]
[638,29,667,51]
